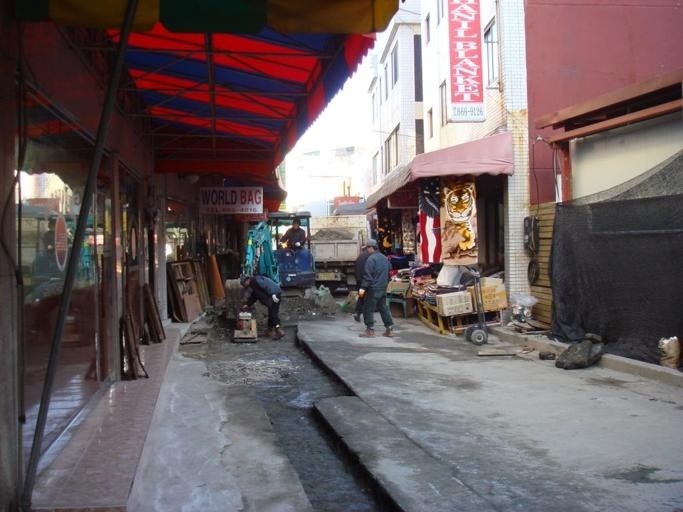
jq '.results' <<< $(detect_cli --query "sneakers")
[353,313,360,321]
[263,329,285,340]
[359,330,374,338]
[383,331,393,336]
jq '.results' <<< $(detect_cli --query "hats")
[240,276,246,285]
[361,239,378,248]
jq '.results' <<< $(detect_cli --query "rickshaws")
[465,265,488,345]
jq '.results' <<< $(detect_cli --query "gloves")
[272,294,279,303]
[359,288,366,298]
[295,242,300,247]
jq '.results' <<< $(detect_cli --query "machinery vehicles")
[17,208,93,284]
[233,211,318,344]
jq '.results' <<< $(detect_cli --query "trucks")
[310,212,373,298]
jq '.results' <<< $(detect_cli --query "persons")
[240,274,284,340]
[43,219,73,256]
[276,217,306,251]
[358,238,395,338]
[353,245,371,322]
